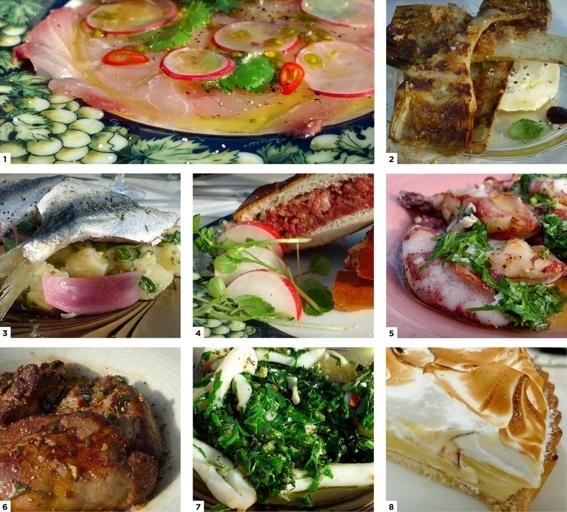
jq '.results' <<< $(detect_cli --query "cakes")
[386,347,562,512]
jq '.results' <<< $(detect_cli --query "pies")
[233,174,374,254]
